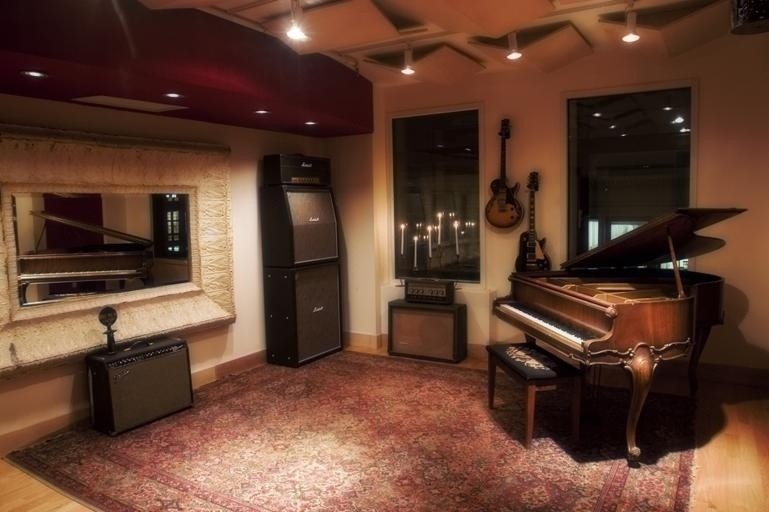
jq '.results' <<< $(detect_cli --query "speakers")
[259,185,338,267]
[87,336,193,436]
[262,264,342,363]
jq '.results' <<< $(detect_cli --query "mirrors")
[1,180,207,324]
[559,76,699,276]
[382,96,489,295]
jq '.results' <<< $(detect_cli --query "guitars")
[485,117,524,228]
[516,171,552,278]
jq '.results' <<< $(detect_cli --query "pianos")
[492,207,747,459]
[17,209,153,305]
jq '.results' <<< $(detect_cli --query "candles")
[398,208,476,270]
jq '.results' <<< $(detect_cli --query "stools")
[484,339,589,450]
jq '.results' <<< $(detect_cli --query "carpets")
[4,347,694,512]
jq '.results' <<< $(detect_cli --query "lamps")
[285,0,311,43]
[357,1,648,78]
